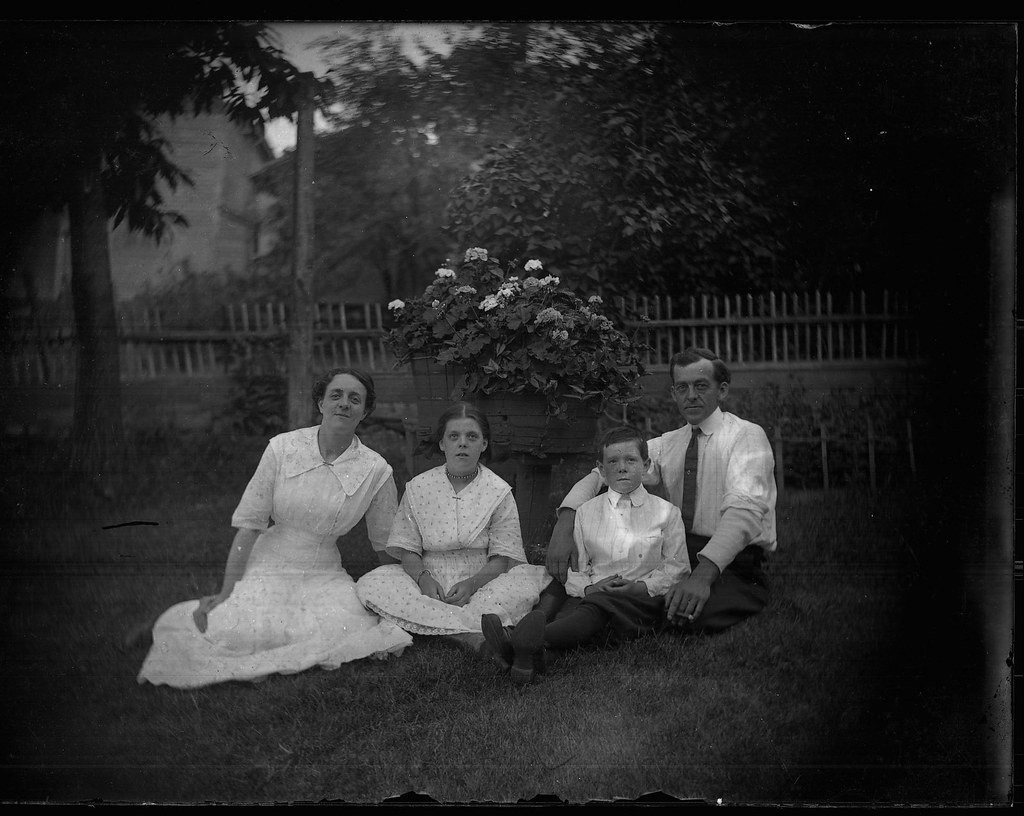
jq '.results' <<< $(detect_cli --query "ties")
[681,427,702,533]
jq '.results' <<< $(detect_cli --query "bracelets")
[416,570,430,583]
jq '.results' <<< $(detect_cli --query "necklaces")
[445,468,477,478]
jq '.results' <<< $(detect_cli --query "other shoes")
[510,609,547,685]
[482,613,517,673]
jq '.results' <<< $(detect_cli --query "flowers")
[383,244,657,421]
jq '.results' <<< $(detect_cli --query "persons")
[483,347,776,684]
[356,403,553,650]
[134,367,413,688]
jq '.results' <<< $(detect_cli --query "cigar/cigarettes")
[674,612,694,620]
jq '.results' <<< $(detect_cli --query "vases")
[479,390,595,454]
